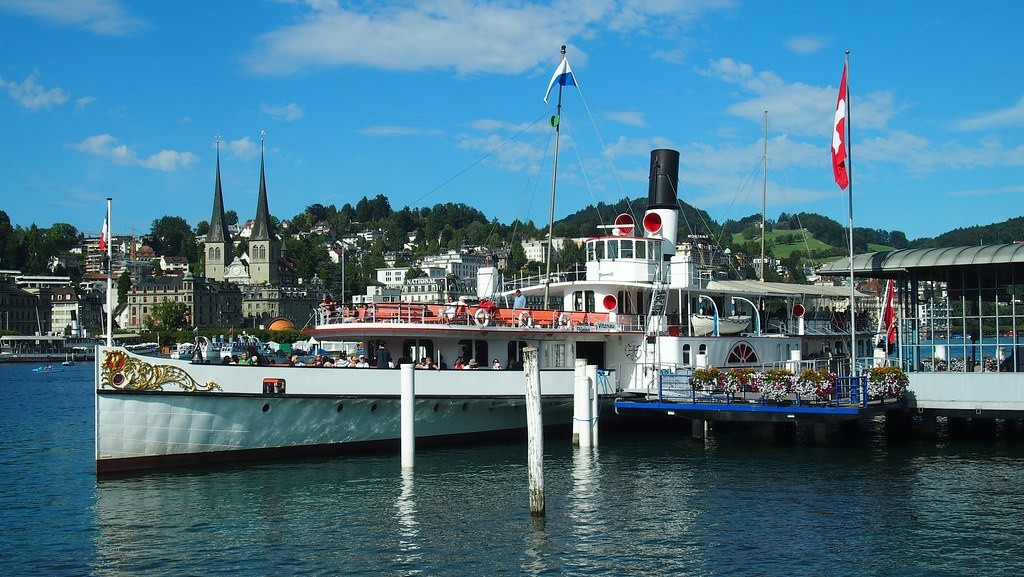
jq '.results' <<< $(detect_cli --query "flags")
[193,327,197,333]
[226,328,232,335]
[544,57,578,104]
[830,61,848,190]
[99,209,108,251]
[884,279,895,344]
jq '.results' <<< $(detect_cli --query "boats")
[0,332,141,361]
[61,360,76,365]
[124,343,161,357]
[194,336,223,361]
[73,346,87,352]
[220,331,316,364]
[94,148,832,481]
[691,313,753,335]
[33,365,64,372]
[170,342,196,359]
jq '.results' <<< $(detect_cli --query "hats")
[359,355,364,358]
[469,358,477,366]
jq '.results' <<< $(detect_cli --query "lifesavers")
[559,313,572,331]
[846,321,852,333]
[475,308,490,327]
[519,311,532,329]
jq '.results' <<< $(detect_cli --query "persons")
[319,296,377,323]
[1001,347,1020,372]
[219,341,522,370]
[513,290,526,308]
[437,295,497,327]
[762,305,869,332]
